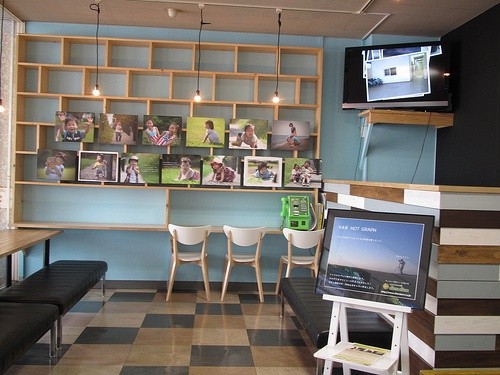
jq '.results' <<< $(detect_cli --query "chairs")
[276,228,326,295]
[166,224,211,302]
[221,224,268,302]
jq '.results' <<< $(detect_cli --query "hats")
[56,152,66,161]
[128,156,138,163]
[210,158,224,167]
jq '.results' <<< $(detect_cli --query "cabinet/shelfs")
[11,33,324,235]
[313,295,414,375]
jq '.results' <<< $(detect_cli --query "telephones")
[280,195,312,230]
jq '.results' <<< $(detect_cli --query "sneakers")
[231,141,241,147]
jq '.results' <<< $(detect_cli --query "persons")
[210,159,236,182]
[121,153,126,171]
[202,120,219,144]
[400,259,405,273]
[145,118,181,145]
[256,162,270,178]
[126,156,140,183]
[232,124,257,148]
[178,157,200,180]
[45,152,66,179]
[92,154,107,180]
[287,123,301,146]
[292,161,313,184]
[114,121,122,142]
[58,113,93,142]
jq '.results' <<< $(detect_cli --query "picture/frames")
[243,156,282,188]
[78,150,119,182]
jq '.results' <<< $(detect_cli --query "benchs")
[280,278,393,375]
[0,259,108,375]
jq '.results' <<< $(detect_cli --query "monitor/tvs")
[342,41,449,110]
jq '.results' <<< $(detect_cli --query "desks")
[0,230,64,288]
